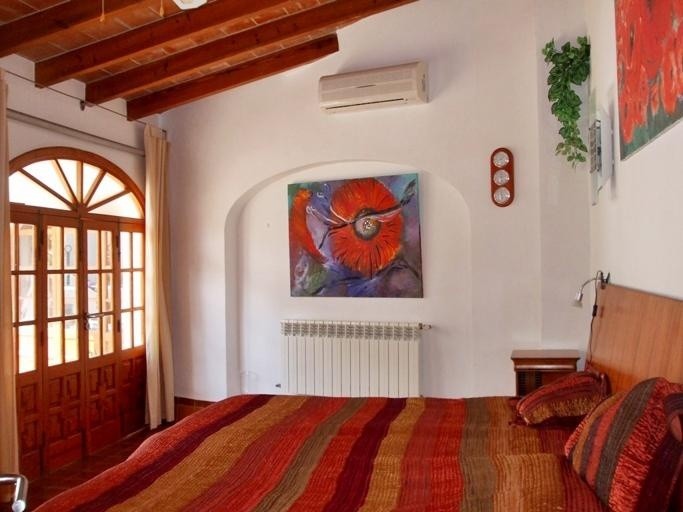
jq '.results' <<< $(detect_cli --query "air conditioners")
[319,60,428,116]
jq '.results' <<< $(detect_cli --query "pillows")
[517,361,607,429]
[567,378,683,511]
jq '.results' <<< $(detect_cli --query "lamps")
[571,271,609,309]
[491,148,514,207]
[173,0,208,11]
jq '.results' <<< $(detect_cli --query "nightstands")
[511,349,581,398]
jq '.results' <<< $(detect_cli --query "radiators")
[280,319,432,397]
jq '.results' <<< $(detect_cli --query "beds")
[32,282,682,511]
